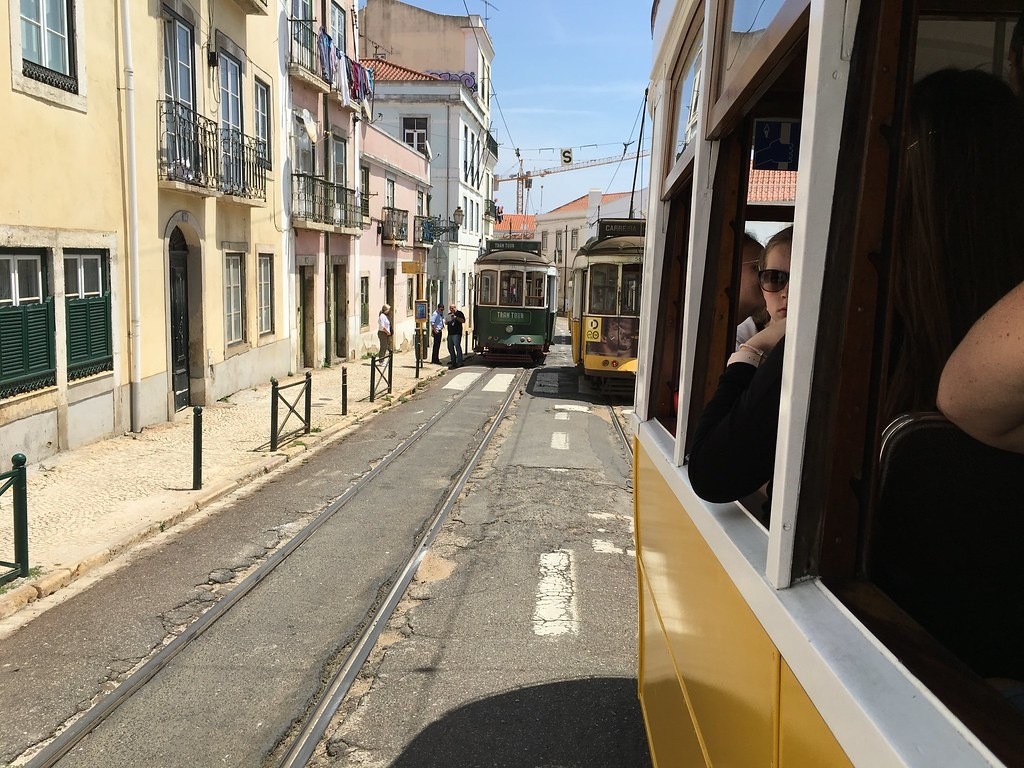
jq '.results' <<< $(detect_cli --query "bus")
[630,0,1024,768]
[567,219,646,391]
[467,238,560,363]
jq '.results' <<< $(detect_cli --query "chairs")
[860,411,1024,680]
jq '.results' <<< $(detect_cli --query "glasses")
[758,269,790,292]
[438,308,444,311]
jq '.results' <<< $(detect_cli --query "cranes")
[494,144,650,214]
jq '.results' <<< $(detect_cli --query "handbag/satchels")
[390,325,394,336]
[431,329,435,337]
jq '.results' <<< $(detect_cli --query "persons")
[377,304,391,366]
[430,304,445,365]
[445,304,466,367]
[687,12,1024,501]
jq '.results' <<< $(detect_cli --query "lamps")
[424,206,465,237]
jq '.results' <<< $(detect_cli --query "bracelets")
[740,344,767,358]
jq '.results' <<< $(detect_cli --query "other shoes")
[380,361,389,366]
[447,361,456,364]
[432,360,442,364]
